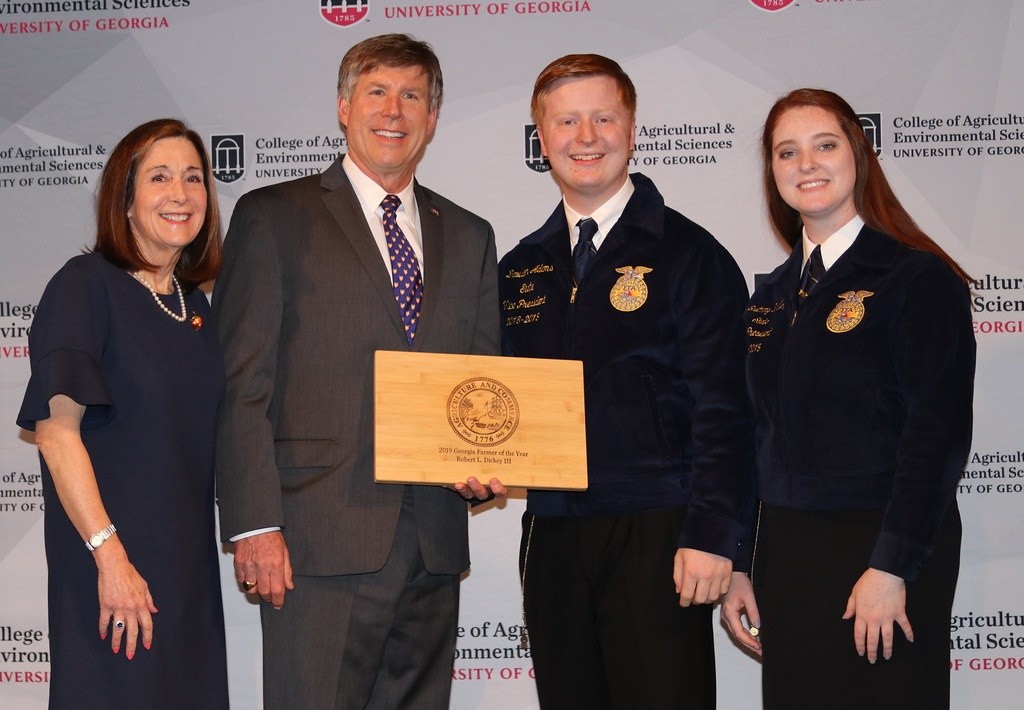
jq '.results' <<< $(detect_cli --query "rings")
[243,580,257,592]
[112,621,125,629]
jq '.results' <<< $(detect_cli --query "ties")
[378,193,424,349]
[571,218,598,294]
[789,244,828,309]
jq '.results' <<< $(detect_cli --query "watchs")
[85,524,117,551]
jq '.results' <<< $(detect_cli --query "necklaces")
[136,272,186,321]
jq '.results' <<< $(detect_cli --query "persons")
[720,89,978,709]
[17,117,232,710]
[487,53,753,709]
[212,32,507,710]
